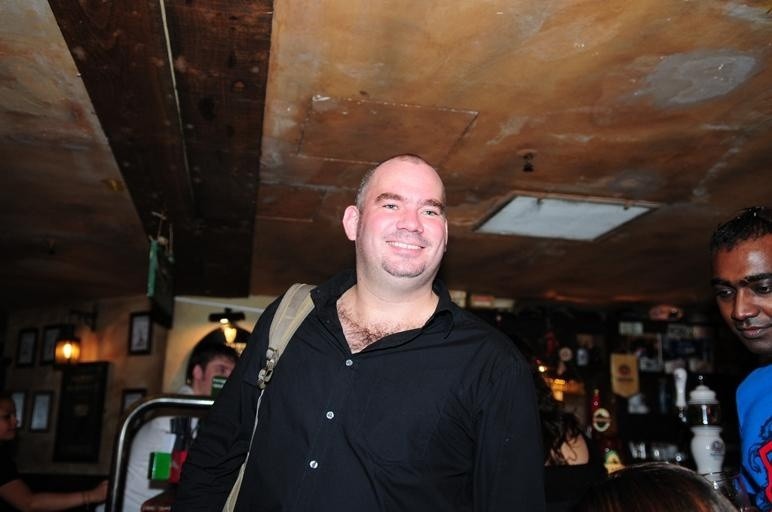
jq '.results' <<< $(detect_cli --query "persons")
[709,203,772,511]
[173,152,548,512]
[0,393,109,512]
[540,410,592,470]
[570,461,746,511]
[96,339,245,512]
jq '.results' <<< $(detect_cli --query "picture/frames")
[12,389,55,431]
[120,387,146,419]
[129,312,152,355]
[17,327,59,367]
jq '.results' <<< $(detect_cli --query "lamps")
[53,323,84,369]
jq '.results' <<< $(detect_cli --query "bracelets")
[81,488,89,507]
